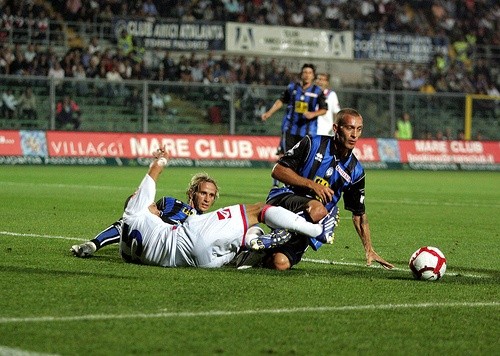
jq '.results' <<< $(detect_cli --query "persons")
[263,64,328,187]
[0,0,500,140]
[70,108,395,270]
[316,73,342,134]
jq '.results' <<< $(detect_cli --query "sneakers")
[251,228,292,250]
[315,206,340,244]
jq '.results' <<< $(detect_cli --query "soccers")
[408,245,448,281]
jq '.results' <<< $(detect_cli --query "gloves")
[70,244,94,257]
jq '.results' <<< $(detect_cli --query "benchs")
[0,0,500,143]
